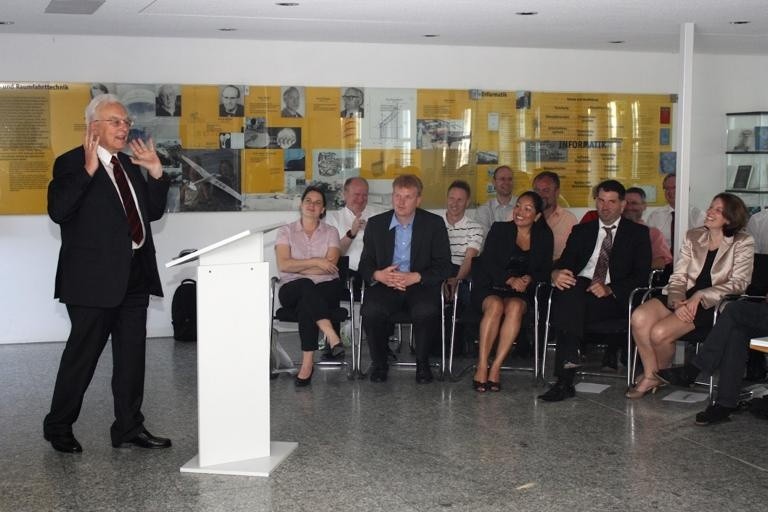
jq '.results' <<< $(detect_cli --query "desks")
[166,223,299,477]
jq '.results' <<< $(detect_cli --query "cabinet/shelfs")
[725,111,768,255]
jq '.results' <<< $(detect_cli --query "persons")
[437,180,484,301]
[340,88,364,118]
[471,191,555,393]
[505,171,577,261]
[42,92,172,453]
[652,302,768,424]
[157,85,180,117]
[273,185,346,386]
[282,87,303,117]
[219,86,244,117]
[474,166,518,228]
[646,174,676,256]
[321,177,381,361]
[625,193,756,398]
[358,174,452,384]
[621,187,672,267]
[537,179,652,403]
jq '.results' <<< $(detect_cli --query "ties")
[667,210,679,256]
[591,225,617,288]
[347,112,353,117]
[106,155,144,246]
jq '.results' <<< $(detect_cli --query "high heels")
[626,378,659,399]
[294,366,315,388]
[328,341,346,358]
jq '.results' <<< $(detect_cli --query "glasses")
[342,94,360,100]
[90,118,136,127]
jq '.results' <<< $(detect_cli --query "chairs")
[267,255,357,381]
[448,257,547,382]
[542,263,653,389]
[631,255,768,387]
[354,261,451,381]
[715,296,768,417]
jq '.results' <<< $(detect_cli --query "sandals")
[471,369,487,393]
[487,366,501,393]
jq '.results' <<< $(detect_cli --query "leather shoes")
[370,359,389,382]
[42,411,84,454]
[537,384,577,404]
[694,404,734,426]
[564,356,585,369]
[415,363,434,385]
[652,366,696,388]
[112,424,172,451]
